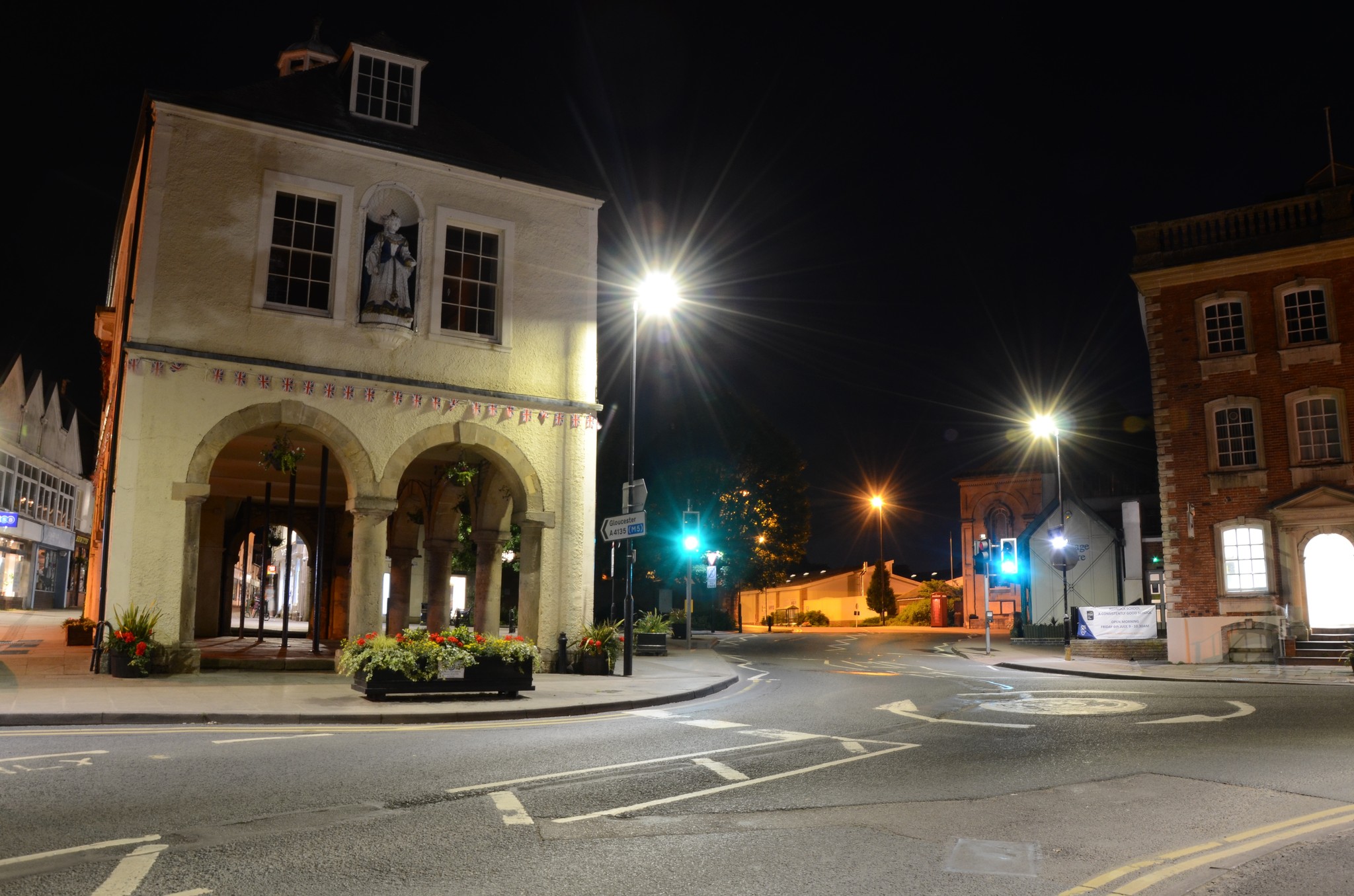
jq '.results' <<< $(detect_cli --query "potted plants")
[633,608,674,657]
[1338,640,1354,673]
[59,617,96,645]
[668,607,687,639]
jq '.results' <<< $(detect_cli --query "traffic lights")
[978,539,991,562]
[1000,538,1017,574]
[682,511,700,558]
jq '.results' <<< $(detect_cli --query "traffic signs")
[599,509,647,542]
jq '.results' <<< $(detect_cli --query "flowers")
[337,624,545,682]
[441,462,479,486]
[259,435,306,475]
[99,599,163,676]
[567,614,624,673]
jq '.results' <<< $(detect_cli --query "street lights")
[620,274,677,676]
[739,536,766,633]
[1032,414,1072,660]
[871,494,886,626]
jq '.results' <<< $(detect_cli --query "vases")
[451,475,468,486]
[351,655,536,702]
[271,456,289,472]
[110,648,143,678]
[579,652,616,675]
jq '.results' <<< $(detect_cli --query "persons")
[365,210,417,319]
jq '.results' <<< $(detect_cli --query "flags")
[234,371,246,386]
[411,394,422,408]
[488,404,498,416]
[151,361,164,377]
[128,359,141,373]
[471,401,481,415]
[323,384,336,398]
[392,391,403,404]
[364,388,375,402]
[343,386,354,400]
[431,396,441,409]
[522,408,531,421]
[282,378,293,392]
[169,362,185,372]
[506,405,514,417]
[257,374,269,389]
[210,368,224,383]
[449,399,458,411]
[540,411,594,428]
